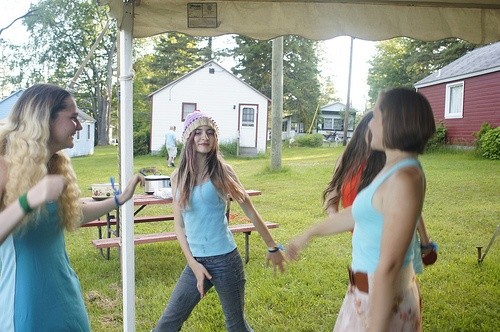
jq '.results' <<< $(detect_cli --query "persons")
[0,84,145,332]
[164,125,182,167]
[285,86,438,332]
[150,112,289,332]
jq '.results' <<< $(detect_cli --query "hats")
[181,110,219,144]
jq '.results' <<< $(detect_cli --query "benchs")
[81,215,175,229]
[91,221,280,249]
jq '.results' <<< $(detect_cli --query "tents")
[93,0,499,332]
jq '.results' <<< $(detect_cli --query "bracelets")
[110,177,124,206]
[268,244,284,252]
[420,241,435,250]
[18,192,34,214]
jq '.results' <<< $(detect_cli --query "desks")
[80,190,262,266]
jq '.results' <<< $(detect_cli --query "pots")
[87,182,120,200]
[144,173,171,195]
